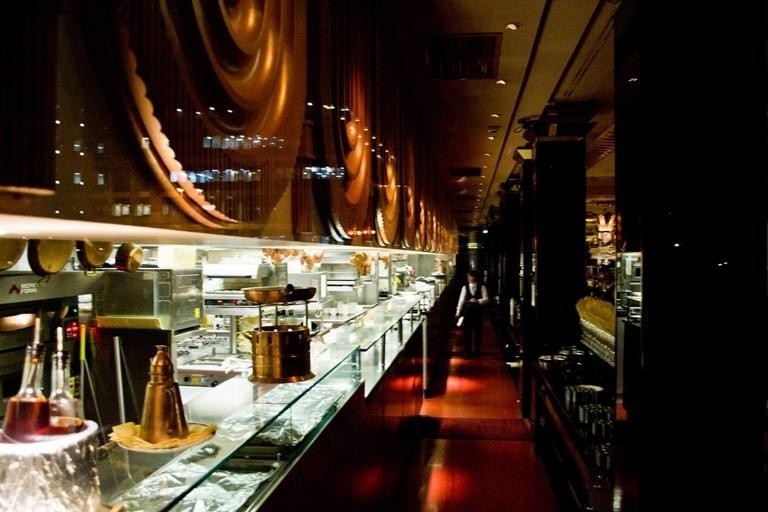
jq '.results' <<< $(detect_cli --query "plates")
[117,423,216,453]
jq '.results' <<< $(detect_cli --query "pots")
[0,240,144,276]
[566,385,615,469]
[243,283,316,304]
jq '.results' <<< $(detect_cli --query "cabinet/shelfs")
[0,212,459,512]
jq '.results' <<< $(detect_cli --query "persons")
[454,269,490,359]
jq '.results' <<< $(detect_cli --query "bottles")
[4,341,82,435]
